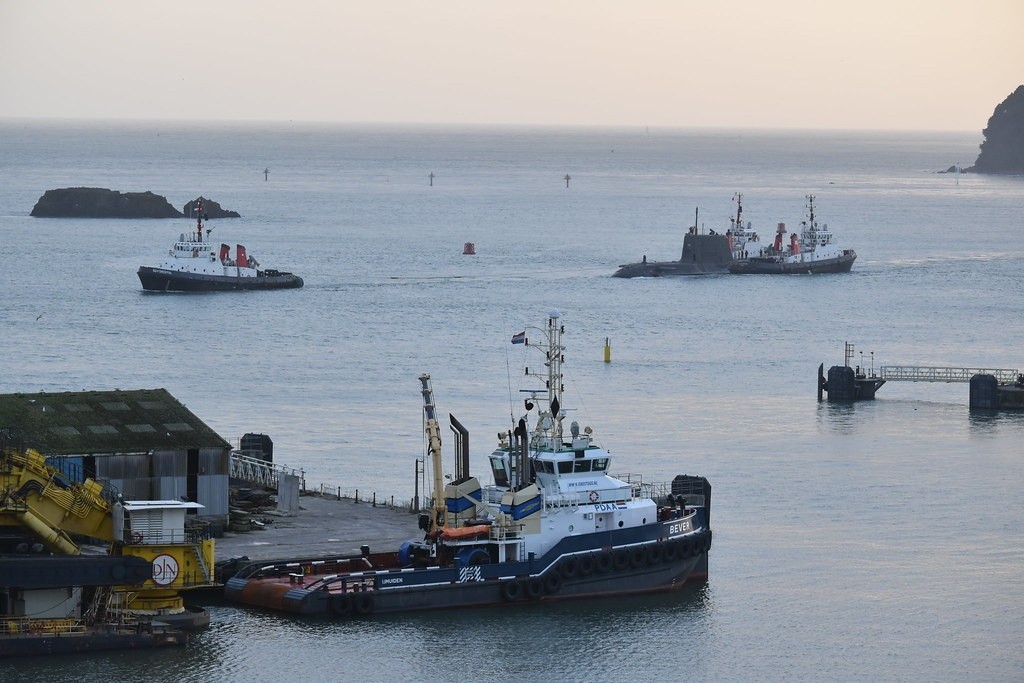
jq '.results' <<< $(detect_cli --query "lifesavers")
[649,529,712,562]
[613,550,629,569]
[589,491,599,503]
[504,580,522,601]
[542,570,563,593]
[578,556,596,575]
[560,557,580,578]
[527,579,544,598]
[630,548,647,566]
[594,552,613,573]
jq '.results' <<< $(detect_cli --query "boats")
[0,448,224,658]
[223,309,714,617]
[136,197,305,293]
[612,192,860,279]
